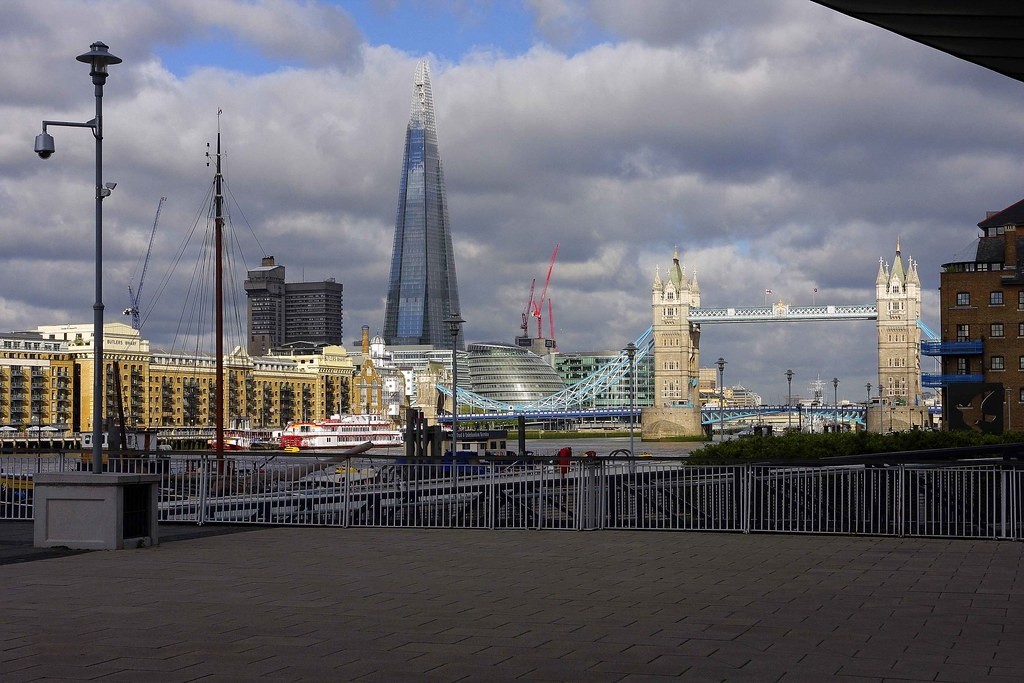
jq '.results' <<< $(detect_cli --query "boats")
[0,106,378,509]
[280,399,404,448]
[393,406,577,480]
[640,454,652,457]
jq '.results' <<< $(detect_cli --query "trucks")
[704,404,718,407]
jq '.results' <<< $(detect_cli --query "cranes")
[532,243,560,339]
[520,278,536,337]
[124,196,167,329]
[548,297,558,351]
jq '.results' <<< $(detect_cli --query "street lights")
[784,369,796,435]
[831,378,840,434]
[842,404,849,435]
[714,357,728,442]
[1005,387,1012,430]
[877,385,885,437]
[34,41,123,475]
[442,312,467,489]
[864,383,873,434]
[811,403,818,434]
[622,342,640,474]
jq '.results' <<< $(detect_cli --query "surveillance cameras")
[35,136,56,159]
